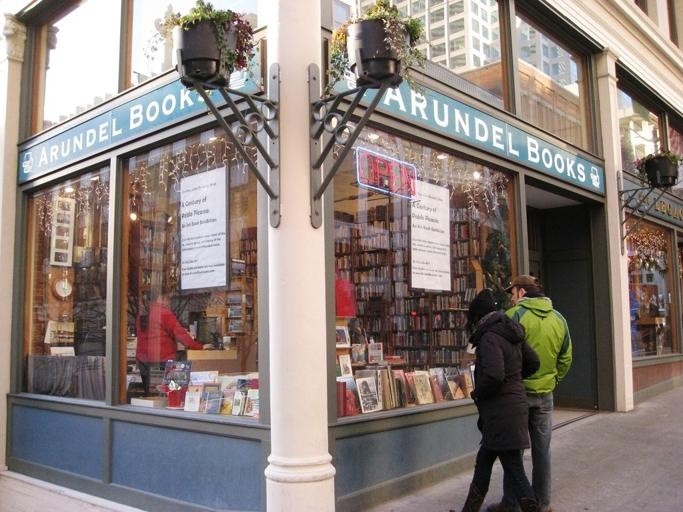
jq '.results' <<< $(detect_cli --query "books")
[137,220,269,337]
[337,347,407,418]
[406,365,474,405]
[132,397,169,406]
[163,360,259,418]
[331,188,471,344]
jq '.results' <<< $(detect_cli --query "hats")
[467,289,495,320]
[504,274,538,293]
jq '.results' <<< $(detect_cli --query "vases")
[647,157,677,186]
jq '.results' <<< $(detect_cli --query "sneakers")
[486,502,519,511]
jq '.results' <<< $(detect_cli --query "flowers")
[634,145,681,172]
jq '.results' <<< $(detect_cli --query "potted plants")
[323,0,435,96]
[164,0,257,89]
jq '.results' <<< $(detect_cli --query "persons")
[484,276,572,511]
[449,290,543,512]
[133,282,216,393]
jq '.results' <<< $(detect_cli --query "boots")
[518,496,542,511]
[450,483,488,512]
